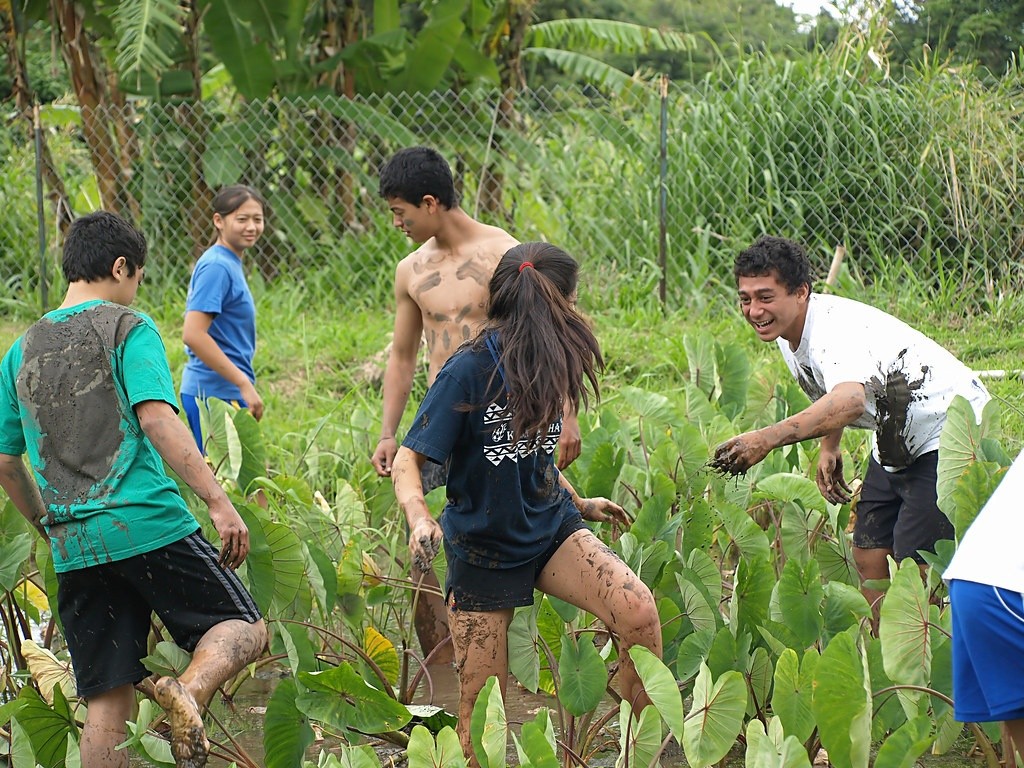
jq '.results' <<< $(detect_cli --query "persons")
[0,182,267,768]
[372,144,663,768]
[710,234,992,642]
[941,444,1024,768]
